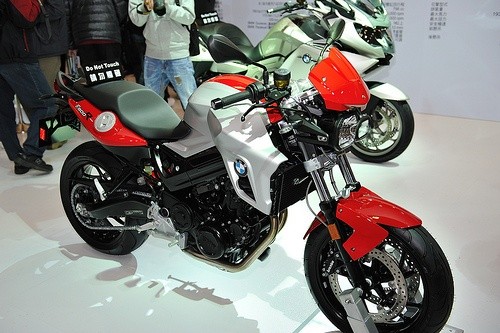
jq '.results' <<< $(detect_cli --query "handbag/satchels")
[19,0,69,59]
[175,0,199,56]
[65,56,84,78]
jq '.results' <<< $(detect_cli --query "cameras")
[153,0,166,16]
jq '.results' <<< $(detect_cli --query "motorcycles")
[188,0,417,164]
[54,18,455,333]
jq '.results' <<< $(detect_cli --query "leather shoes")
[13,164,31,175]
[13,151,53,172]
